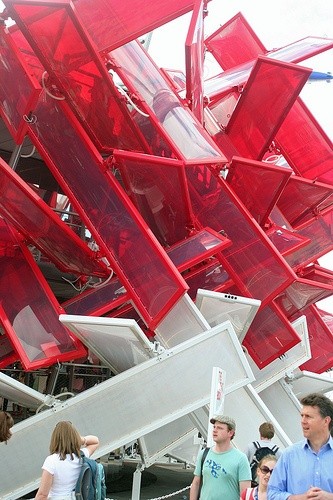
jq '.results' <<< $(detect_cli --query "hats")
[210,415,235,430]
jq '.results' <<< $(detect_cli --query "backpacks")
[76,449,106,500]
[250,441,280,486]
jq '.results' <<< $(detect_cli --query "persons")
[247,423,282,489]
[190,416,252,500]
[240,454,278,500]
[266,393,333,500]
[34,421,100,500]
[2,347,116,419]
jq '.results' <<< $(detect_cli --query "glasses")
[258,465,274,475]
